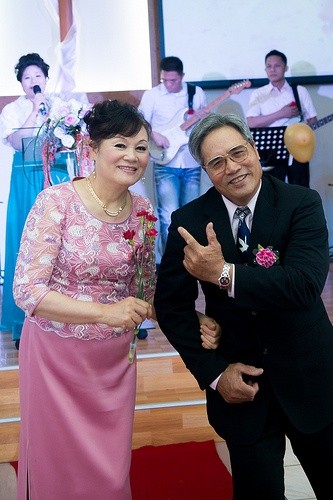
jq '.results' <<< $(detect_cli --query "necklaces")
[86,178,126,217]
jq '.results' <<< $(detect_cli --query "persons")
[0,52,83,348]
[136,57,211,251]
[245,50,318,189]
[13,100,221,500]
[153,113,333,500]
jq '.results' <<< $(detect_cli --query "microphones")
[33,85,46,116]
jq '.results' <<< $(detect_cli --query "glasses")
[159,79,176,84]
[203,140,248,175]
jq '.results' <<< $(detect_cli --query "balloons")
[284,122,316,162]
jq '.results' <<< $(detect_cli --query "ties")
[235,207,252,258]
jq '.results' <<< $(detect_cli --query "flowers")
[122,208,159,365]
[48,98,94,148]
[254,243,279,269]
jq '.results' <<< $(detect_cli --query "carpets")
[10,439,233,500]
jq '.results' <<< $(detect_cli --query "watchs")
[218,262,231,289]
